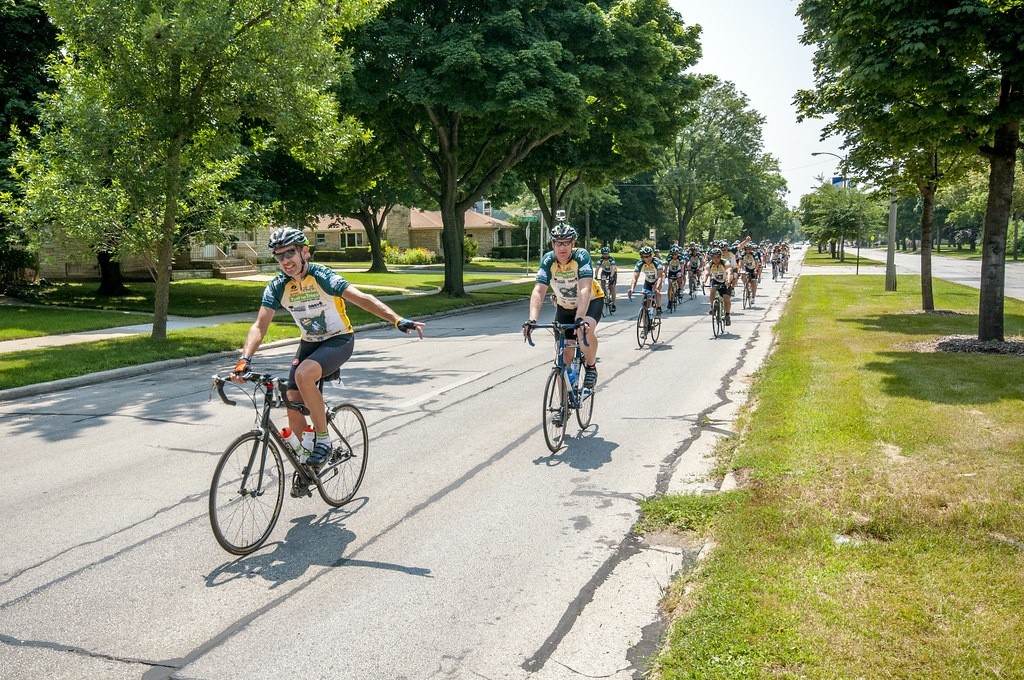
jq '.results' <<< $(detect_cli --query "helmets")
[666,240,788,262]
[654,249,659,254]
[600,247,610,253]
[268,227,308,254]
[550,223,577,241]
[639,246,653,257]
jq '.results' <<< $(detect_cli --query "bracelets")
[239,356,251,361]
[395,318,402,329]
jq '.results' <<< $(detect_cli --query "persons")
[653,237,791,309]
[230,228,426,498]
[629,246,663,339]
[595,247,617,312]
[522,223,604,424]
[702,248,733,326]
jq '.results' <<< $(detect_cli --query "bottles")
[572,358,580,386]
[300,425,315,464]
[648,307,655,320]
[280,427,304,456]
[567,367,576,385]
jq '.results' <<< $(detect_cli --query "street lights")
[812,151,847,261]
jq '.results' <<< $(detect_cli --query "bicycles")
[628,289,661,348]
[701,250,790,309]
[594,277,615,318]
[664,269,685,314]
[684,268,705,300]
[521,318,595,453]
[208,366,369,556]
[701,283,735,338]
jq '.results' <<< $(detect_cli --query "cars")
[793,242,802,250]
[806,241,810,245]
[799,241,804,245]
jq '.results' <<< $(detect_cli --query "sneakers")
[306,443,333,466]
[552,411,570,424]
[657,309,663,317]
[612,304,616,312]
[583,368,598,388]
[725,315,730,326]
[640,329,646,338]
[709,310,715,316]
[290,475,310,497]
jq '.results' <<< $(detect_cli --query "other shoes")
[679,291,683,298]
[667,301,671,308]
[751,298,755,304]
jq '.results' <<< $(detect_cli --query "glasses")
[722,249,726,251]
[553,240,572,247]
[274,248,299,262]
[745,249,751,250]
